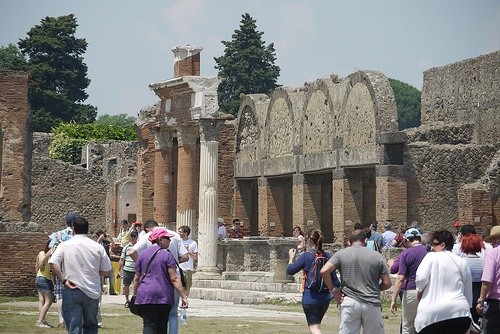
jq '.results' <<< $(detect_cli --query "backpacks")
[304,251,333,294]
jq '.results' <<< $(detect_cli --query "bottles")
[181,307,187,325]
[482,301,489,315]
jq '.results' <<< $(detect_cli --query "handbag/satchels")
[179,268,186,286]
[129,296,144,315]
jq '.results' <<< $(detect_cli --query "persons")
[48,217,113,334]
[35,212,247,328]
[319,229,393,334]
[286,229,341,334]
[475,226,500,334]
[413,229,481,334]
[131,227,189,334]
[391,228,431,334]
[290,222,494,334]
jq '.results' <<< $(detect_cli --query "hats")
[147,227,175,241]
[371,223,377,230]
[404,228,422,238]
[132,221,142,224]
[484,226,500,242]
[218,218,224,223]
[131,231,137,235]
[66,213,75,223]
[384,222,392,227]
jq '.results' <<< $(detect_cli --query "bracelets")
[182,295,188,299]
[62,280,67,285]
[477,299,484,304]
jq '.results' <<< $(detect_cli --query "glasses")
[131,235,136,237]
[234,223,241,225]
[135,225,141,227]
[432,243,442,247]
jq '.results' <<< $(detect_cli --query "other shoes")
[111,292,116,295]
[37,320,50,328]
[125,300,130,308]
